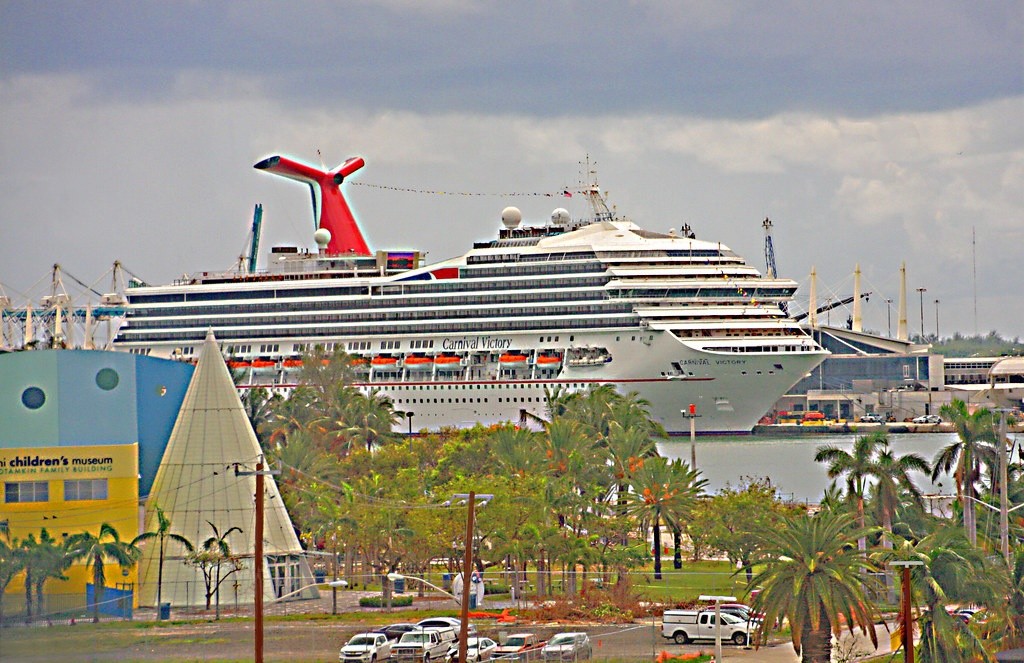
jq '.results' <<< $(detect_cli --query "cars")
[860,413,882,422]
[912,415,943,423]
[370,617,476,649]
[446,638,497,663]
[541,633,592,662]
[699,603,778,630]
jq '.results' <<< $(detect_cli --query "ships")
[101,152,832,436]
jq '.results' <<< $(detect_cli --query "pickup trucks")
[391,630,450,663]
[661,611,761,644]
[340,633,397,663]
[490,633,548,658]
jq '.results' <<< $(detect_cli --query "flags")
[564,191,572,197]
